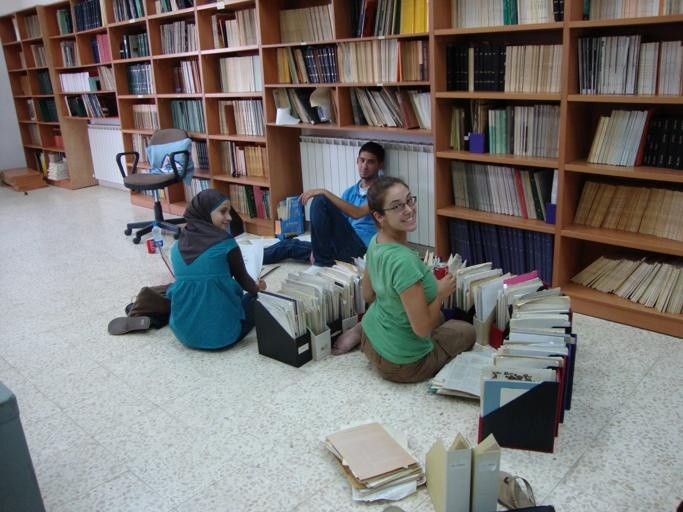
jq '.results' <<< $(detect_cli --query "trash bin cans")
[0,383,45,512]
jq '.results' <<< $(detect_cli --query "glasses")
[379,195,417,214]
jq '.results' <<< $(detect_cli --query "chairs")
[115,129,194,243]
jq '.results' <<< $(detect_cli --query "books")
[19,14,68,180]
[421,250,577,419]
[569,0,681,314]
[255,254,369,343]
[56,0,117,118]
[155,0,210,202]
[271,0,432,133]
[114,1,165,198]
[447,2,563,286]
[212,9,270,221]
[320,418,426,506]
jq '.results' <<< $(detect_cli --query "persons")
[261,141,385,266]
[331,176,474,385]
[107,189,267,351]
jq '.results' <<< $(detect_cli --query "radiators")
[299,134,435,249]
[86,124,128,184]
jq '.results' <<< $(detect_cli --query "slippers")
[496,468,537,510]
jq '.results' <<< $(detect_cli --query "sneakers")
[107,314,153,336]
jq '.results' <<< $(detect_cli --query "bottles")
[151,223,164,253]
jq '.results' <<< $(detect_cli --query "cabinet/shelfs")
[146,0,213,226]
[257,0,431,149]
[102,0,168,203]
[565,0,682,340]
[196,0,299,224]
[429,0,561,290]
[0,0,98,190]
[38,0,120,121]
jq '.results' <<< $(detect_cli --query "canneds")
[433,264,449,280]
[146,239,155,254]
